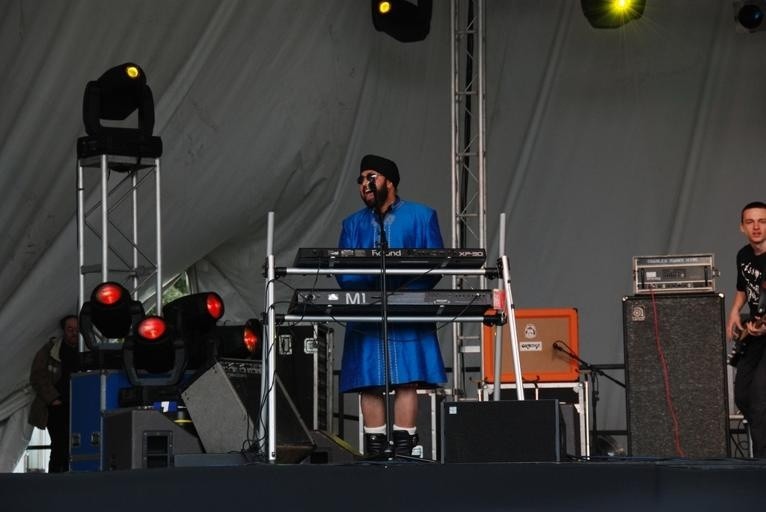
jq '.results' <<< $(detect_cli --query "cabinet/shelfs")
[77,156,162,354]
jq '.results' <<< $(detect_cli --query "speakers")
[103,409,203,471]
[216,321,334,435]
[181,360,317,464]
[440,399,566,462]
[622,292,731,460]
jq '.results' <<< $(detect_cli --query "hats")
[360,155,400,187]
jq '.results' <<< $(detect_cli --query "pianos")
[288,288,493,315]
[292,248,486,269]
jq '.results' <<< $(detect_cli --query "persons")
[335,156,443,464]
[30,315,90,473]
[725,201,766,456]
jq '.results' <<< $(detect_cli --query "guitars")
[726,312,766,366]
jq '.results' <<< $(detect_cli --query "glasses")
[357,172,378,184]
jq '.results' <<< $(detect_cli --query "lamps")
[214,325,259,358]
[82,62,155,136]
[162,291,225,336]
[122,316,185,386]
[581,0,646,30]
[372,0,433,42]
[79,281,144,352]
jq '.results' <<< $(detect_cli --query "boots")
[393,429,419,461]
[362,432,389,460]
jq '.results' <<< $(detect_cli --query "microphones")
[367,173,377,192]
